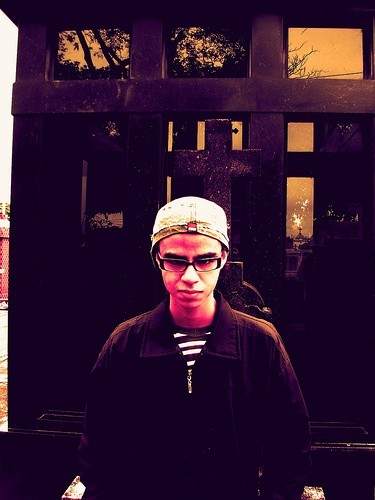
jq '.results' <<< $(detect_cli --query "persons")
[72,196,312,500]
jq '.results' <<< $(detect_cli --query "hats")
[151,196,230,267]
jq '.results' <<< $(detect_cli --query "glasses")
[156,249,222,272]
